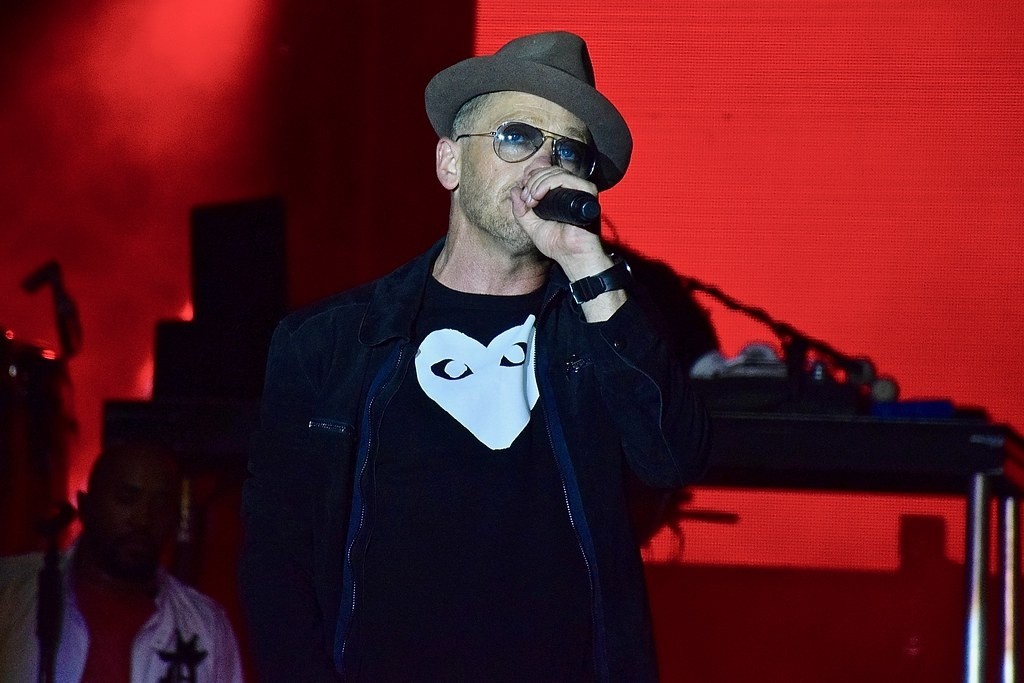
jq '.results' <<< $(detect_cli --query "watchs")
[569,260,634,304]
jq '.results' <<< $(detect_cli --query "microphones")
[522,186,601,227]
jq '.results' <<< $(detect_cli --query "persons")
[0,442,243,683]
[245,31,704,683]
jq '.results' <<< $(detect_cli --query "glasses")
[454,121,601,180]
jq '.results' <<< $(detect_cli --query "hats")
[424,29,633,192]
